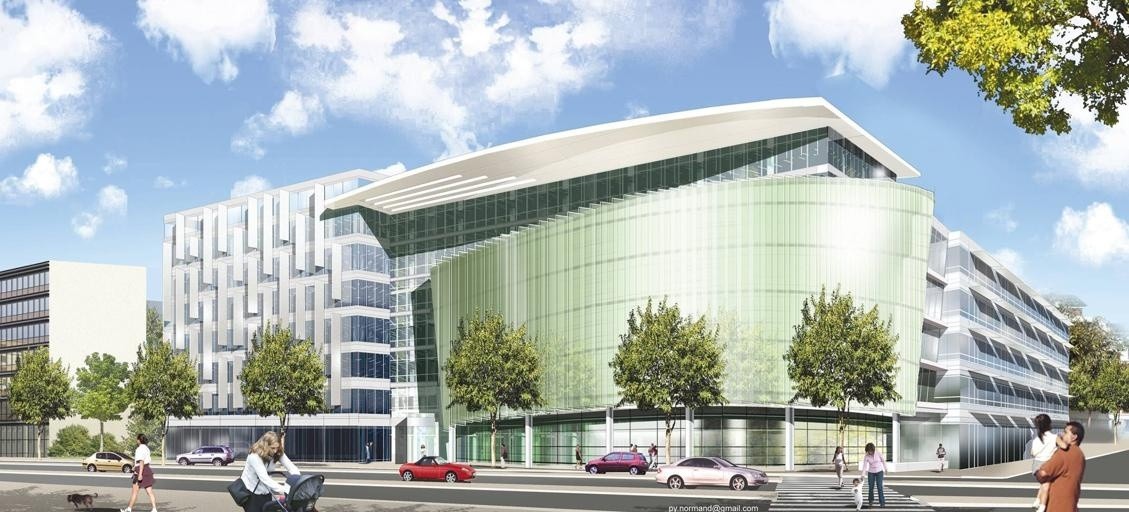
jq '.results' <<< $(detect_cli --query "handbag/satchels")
[228,478,252,506]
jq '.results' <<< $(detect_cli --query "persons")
[499,443,509,469]
[237,430,302,512]
[861,442,888,508]
[630,442,658,470]
[364,442,372,464]
[1035,421,1086,512]
[852,477,864,511]
[574,443,585,469]
[832,445,849,490]
[119,432,160,512]
[1030,413,1068,512]
[418,444,427,459]
[936,442,946,471]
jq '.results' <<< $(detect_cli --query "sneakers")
[120,507,131,512]
